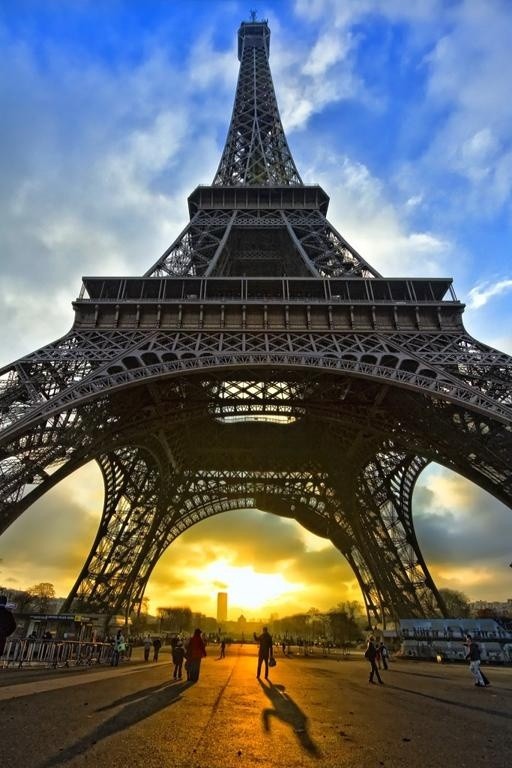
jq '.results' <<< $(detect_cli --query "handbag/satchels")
[269,657,276,665]
[365,649,372,657]
[116,641,126,653]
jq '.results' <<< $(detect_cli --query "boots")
[375,672,384,684]
[369,672,376,684]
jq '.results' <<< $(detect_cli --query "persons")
[367,636,388,685]
[23,631,37,658]
[143,634,153,662]
[465,637,486,687]
[254,626,273,679]
[41,632,52,657]
[462,638,489,685]
[281,643,285,655]
[219,641,225,658]
[171,629,206,682]
[0,595,16,659]
[110,630,124,667]
[153,638,161,662]
